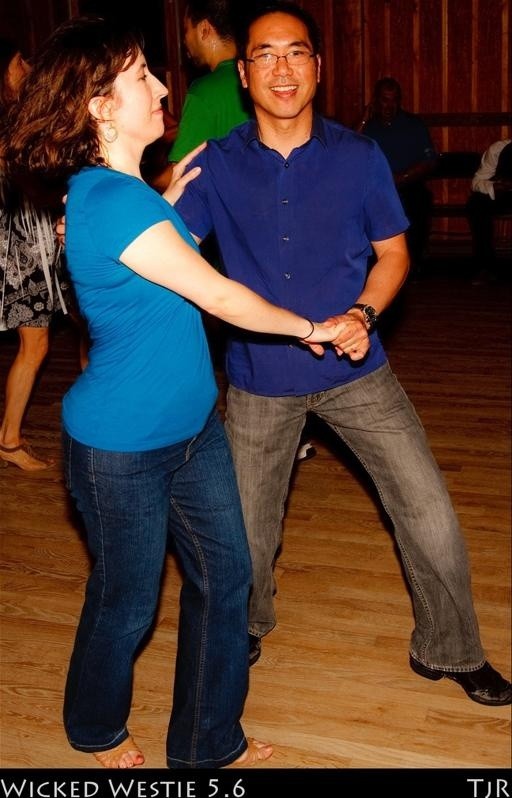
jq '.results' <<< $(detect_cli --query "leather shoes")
[408,652,512,704]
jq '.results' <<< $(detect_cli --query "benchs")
[411,110,509,217]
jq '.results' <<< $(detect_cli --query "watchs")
[351,302,380,329]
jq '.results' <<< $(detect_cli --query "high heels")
[0,442,62,472]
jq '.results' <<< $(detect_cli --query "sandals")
[94,736,145,770]
[231,738,277,766]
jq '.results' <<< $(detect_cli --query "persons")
[0,38,72,470]
[1,14,348,769]
[55,9,511,707]
[361,79,435,230]
[150,3,318,462]
[472,139,512,290]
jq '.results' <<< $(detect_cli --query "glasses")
[244,49,315,70]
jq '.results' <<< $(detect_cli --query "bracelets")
[301,320,315,343]
